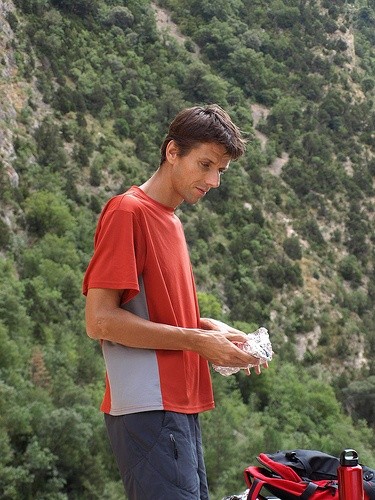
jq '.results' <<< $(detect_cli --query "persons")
[85,104,268,500]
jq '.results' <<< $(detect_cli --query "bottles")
[337,449,364,500]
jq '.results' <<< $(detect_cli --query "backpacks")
[244,449,375,500]
